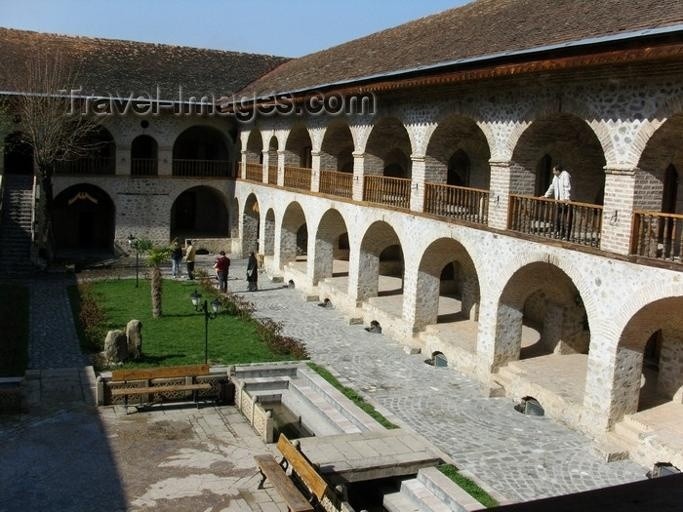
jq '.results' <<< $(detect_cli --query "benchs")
[253,434,329,512]
[111,365,213,415]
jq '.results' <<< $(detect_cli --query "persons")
[245,249,258,292]
[169,239,182,276]
[539,164,573,241]
[183,239,195,280]
[213,251,230,292]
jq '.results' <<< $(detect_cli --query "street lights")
[188,287,223,366]
[125,233,140,288]
